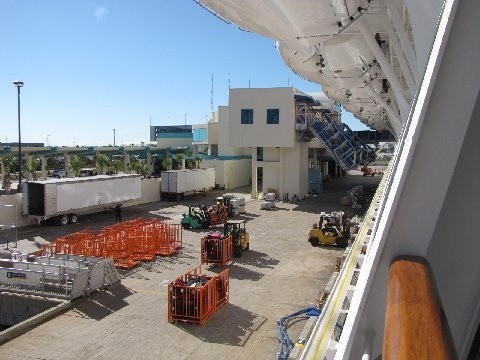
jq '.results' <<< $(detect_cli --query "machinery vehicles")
[181,204,210,229]
[224,220,249,257]
[308,212,350,248]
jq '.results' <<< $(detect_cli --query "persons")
[192,207,202,223]
[232,226,238,238]
[325,220,338,236]
[114,204,124,221]
